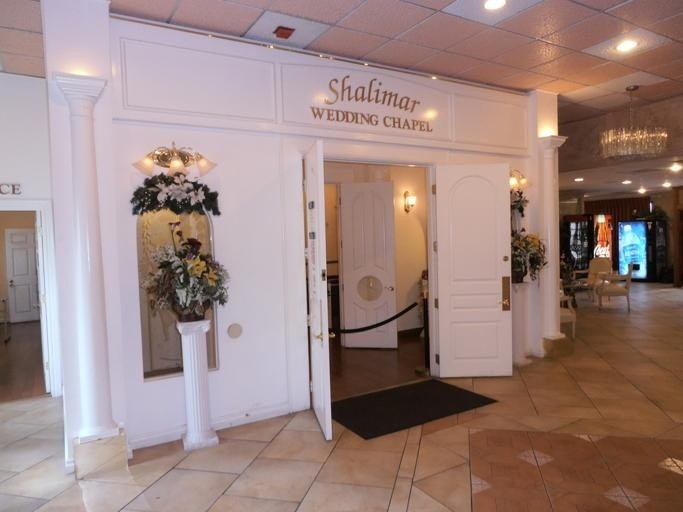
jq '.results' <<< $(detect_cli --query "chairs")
[559,257,633,342]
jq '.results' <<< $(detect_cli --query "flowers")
[129,172,221,216]
[510,189,529,217]
[138,220,230,316]
[510,223,549,282]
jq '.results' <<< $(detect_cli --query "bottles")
[593,214,611,258]
[657,228,666,271]
[622,225,643,277]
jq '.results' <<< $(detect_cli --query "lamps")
[509,168,533,191]
[597,86,667,163]
[131,140,217,179]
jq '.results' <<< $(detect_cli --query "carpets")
[331,377,499,441]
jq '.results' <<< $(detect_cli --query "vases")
[511,267,527,283]
[170,294,210,322]
